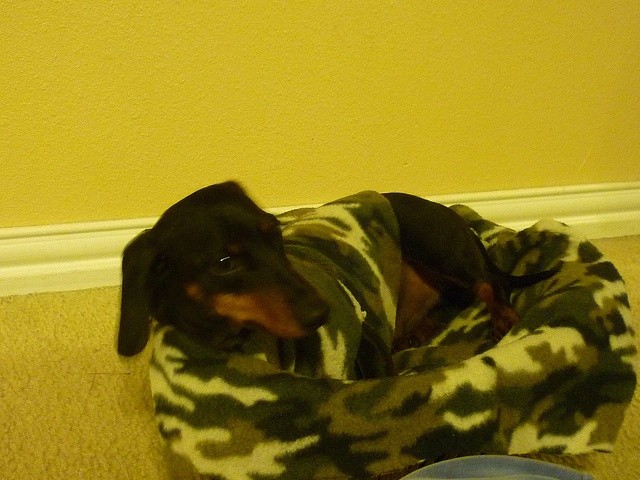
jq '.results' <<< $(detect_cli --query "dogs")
[116,176,520,379]
